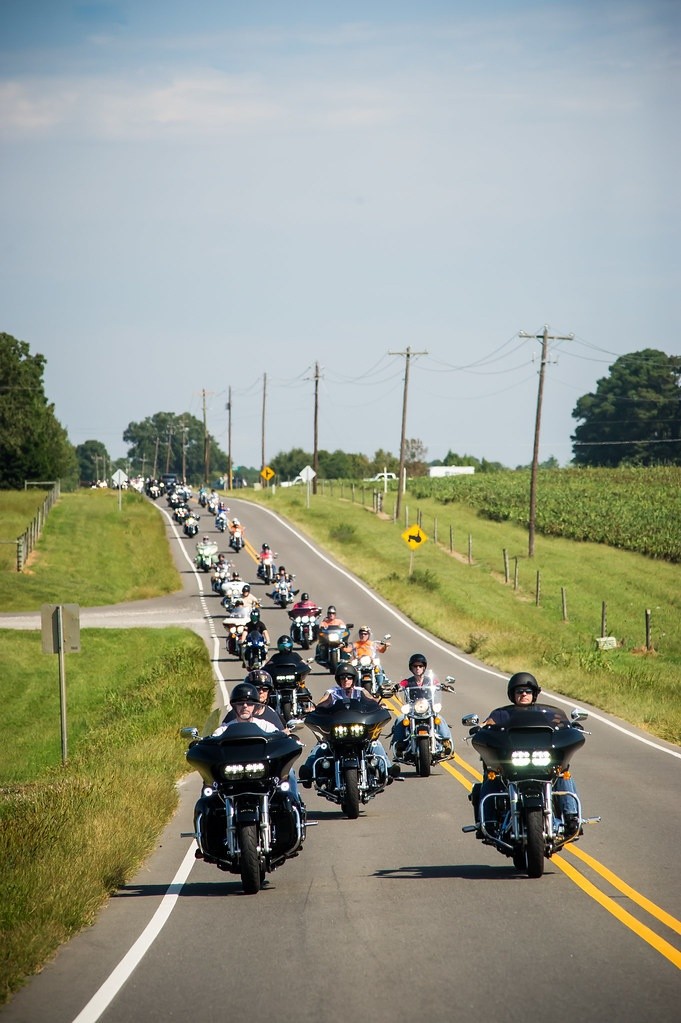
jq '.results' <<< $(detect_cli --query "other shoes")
[382,763,401,786]
[299,765,313,789]
[395,745,403,760]
[442,738,454,755]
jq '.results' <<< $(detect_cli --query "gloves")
[572,722,584,732]
[392,684,401,694]
[469,726,481,735]
[442,686,454,692]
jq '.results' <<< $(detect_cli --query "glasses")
[514,688,534,694]
[237,703,255,706]
[411,665,425,668]
[254,686,270,692]
[340,675,354,680]
[328,612,335,614]
[359,633,368,635]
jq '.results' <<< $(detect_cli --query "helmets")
[230,683,260,710]
[250,610,260,622]
[358,626,372,640]
[327,606,337,616]
[507,672,541,703]
[261,542,309,600]
[170,482,250,596]
[409,654,427,672]
[334,664,358,688]
[244,669,274,689]
[277,635,293,654]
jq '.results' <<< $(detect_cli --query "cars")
[280,476,319,488]
[206,476,242,489]
[371,473,397,482]
[158,474,180,493]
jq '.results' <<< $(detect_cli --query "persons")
[471,673,584,839]
[89,473,452,857]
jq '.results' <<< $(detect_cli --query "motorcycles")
[269,574,297,609]
[296,689,405,819]
[231,596,263,611]
[255,553,279,585]
[211,560,236,597]
[90,474,246,573]
[222,606,252,660]
[461,708,602,879]
[237,630,270,672]
[254,658,315,722]
[221,582,249,613]
[379,675,456,777]
[338,634,391,697]
[288,607,323,649]
[180,719,319,894]
[312,623,354,674]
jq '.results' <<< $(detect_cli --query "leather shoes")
[564,816,584,837]
